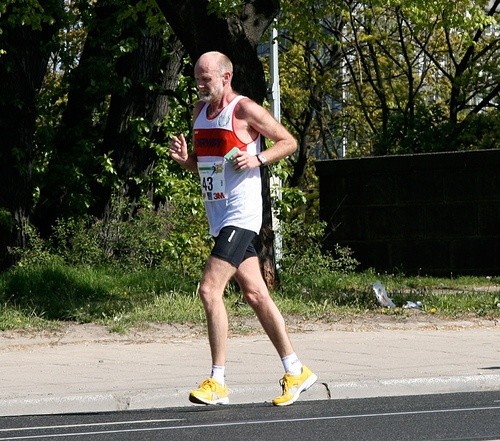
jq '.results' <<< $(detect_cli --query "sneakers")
[272,365,318,407]
[189,378,230,405]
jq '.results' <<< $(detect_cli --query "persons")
[168,51,319,406]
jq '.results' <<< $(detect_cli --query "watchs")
[255,153,268,165]
